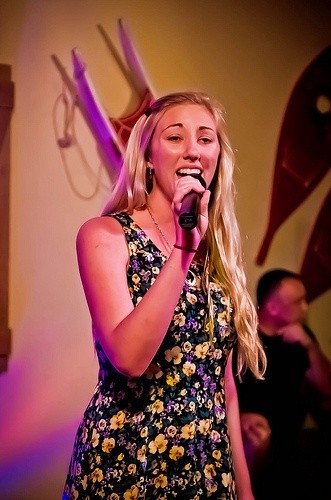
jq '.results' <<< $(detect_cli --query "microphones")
[178,173,206,230]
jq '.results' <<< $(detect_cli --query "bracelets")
[173,244,198,253]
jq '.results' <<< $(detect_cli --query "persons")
[61,94,267,499]
[232,269,331,500]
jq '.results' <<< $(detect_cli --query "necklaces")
[145,204,201,280]
